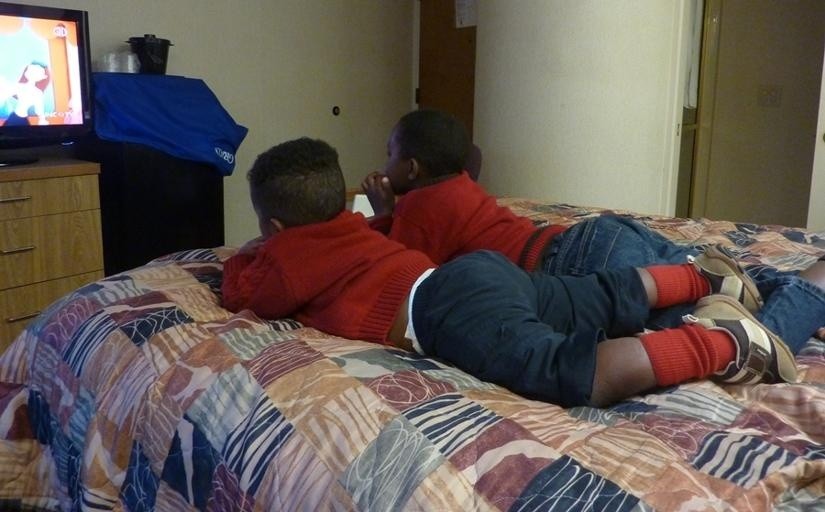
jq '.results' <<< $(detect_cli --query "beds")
[0,198,825,512]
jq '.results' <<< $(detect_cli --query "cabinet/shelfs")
[0,157,106,358]
[90,72,225,276]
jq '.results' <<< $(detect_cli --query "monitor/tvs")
[0,1,91,165]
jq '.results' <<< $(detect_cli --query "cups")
[103,52,140,73]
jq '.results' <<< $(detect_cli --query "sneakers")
[682,247,799,385]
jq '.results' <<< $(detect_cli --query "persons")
[356,104,824,359]
[219,135,800,409]
